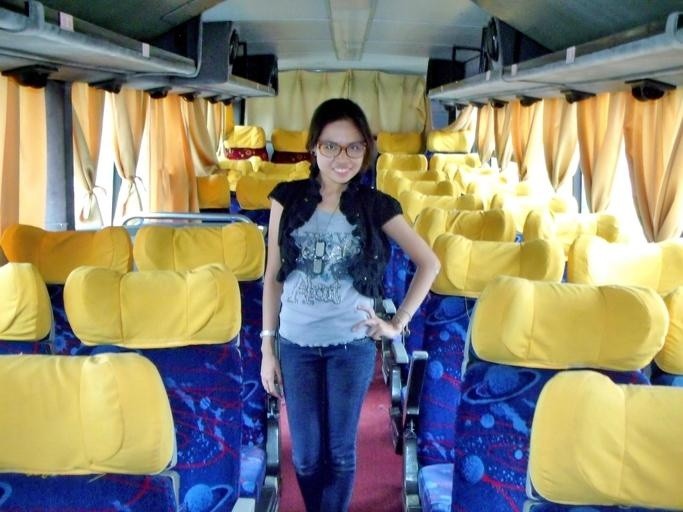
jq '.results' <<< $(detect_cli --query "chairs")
[1,125,314,511]
[372,127,682,511]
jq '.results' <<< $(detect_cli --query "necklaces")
[313,203,338,275]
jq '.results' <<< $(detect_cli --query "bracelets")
[260,330,278,337]
[399,305,414,322]
[395,314,407,330]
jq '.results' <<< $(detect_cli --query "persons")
[259,100,441,512]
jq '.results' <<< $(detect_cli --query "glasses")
[316,141,367,158]
